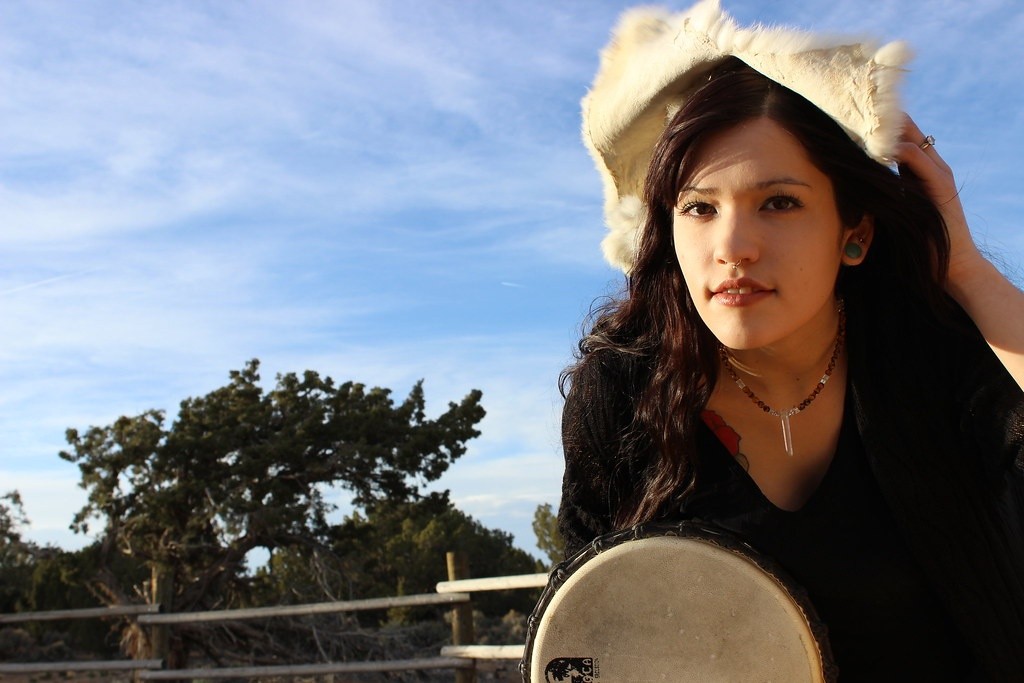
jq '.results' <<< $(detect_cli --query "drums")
[520,522,825,681]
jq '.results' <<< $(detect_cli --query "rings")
[920,135,936,150]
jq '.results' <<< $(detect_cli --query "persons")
[555,1,1024,683]
[893,108,1024,355]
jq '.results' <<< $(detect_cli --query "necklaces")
[717,294,845,455]
